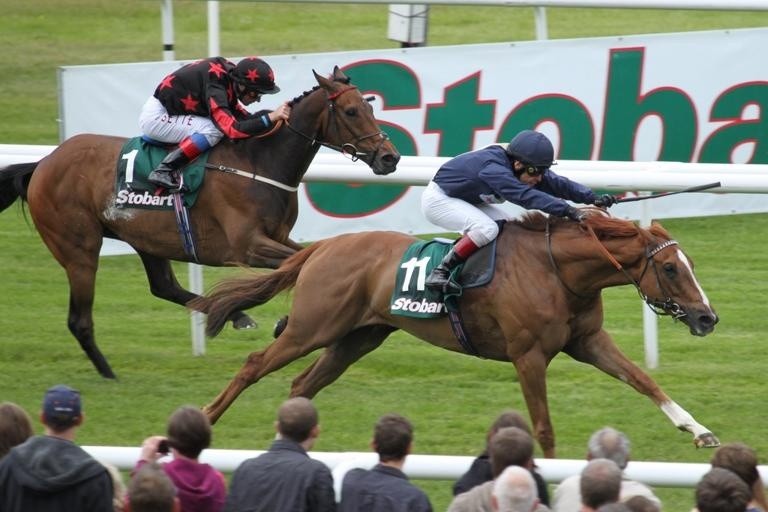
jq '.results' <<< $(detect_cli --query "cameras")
[158,442,170,454]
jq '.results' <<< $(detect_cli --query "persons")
[139,55,291,192]
[421,130,619,292]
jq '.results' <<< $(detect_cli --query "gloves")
[594,193,619,208]
[563,204,588,223]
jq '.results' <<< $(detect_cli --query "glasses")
[527,164,547,175]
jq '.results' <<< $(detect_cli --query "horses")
[184,204,722,460]
[0,63,403,383]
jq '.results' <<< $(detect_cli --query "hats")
[504,129,558,179]
[44,385,81,421]
[229,57,280,108]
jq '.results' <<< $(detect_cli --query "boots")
[147,135,202,189]
[424,233,480,295]
